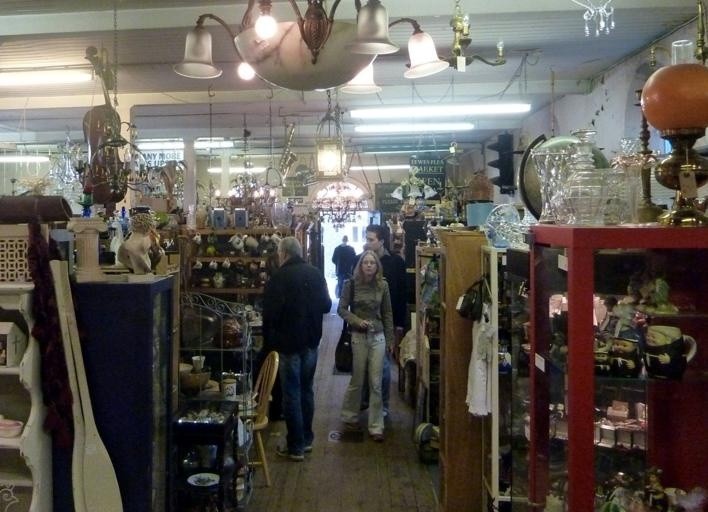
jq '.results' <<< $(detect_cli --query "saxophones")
[279,122,298,178]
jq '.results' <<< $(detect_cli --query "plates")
[187,471,219,488]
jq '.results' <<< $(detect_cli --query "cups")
[611,325,697,381]
[192,230,283,269]
[193,356,205,372]
[252,310,260,321]
[224,380,237,400]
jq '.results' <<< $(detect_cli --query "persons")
[332,235,355,298]
[358,226,409,416]
[351,245,369,272]
[264,235,332,461]
[342,249,395,441]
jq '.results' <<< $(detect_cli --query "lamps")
[0,1,151,195]
[179,99,534,225]
[641,63,708,226]
[172,1,507,95]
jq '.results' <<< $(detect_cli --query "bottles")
[530,126,644,225]
[247,310,252,318]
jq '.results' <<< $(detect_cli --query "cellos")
[83,47,127,203]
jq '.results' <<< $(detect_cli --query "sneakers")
[276,443,313,463]
[344,421,362,433]
[371,434,385,443]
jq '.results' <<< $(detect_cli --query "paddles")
[49,260,124,512]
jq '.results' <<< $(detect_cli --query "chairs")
[247,350,280,488]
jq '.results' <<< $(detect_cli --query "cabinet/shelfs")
[0,284,53,512]
[522,224,707,507]
[438,227,481,510]
[475,243,530,507]
[176,402,239,512]
[178,225,307,296]
[72,277,174,511]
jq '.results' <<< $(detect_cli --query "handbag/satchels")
[335,326,351,372]
[455,289,483,321]
